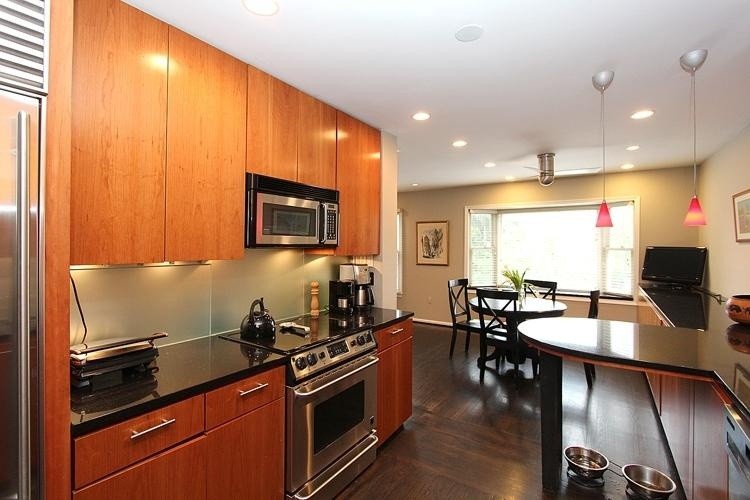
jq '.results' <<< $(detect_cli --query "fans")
[513,152,601,175]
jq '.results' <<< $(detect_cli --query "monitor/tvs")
[641,245,707,288]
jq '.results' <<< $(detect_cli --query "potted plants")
[500,264,531,304]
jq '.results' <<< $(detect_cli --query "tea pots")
[239,339,277,368]
[240,297,276,339]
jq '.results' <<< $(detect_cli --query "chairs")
[581,288,601,389]
[471,288,537,395]
[444,276,502,371]
[522,278,558,358]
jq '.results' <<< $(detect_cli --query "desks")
[511,310,715,500]
[467,295,569,369]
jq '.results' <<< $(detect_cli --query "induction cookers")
[218,313,380,385]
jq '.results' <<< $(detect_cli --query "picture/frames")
[415,220,450,268]
[729,187,750,243]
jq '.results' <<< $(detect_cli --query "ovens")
[284,347,379,500]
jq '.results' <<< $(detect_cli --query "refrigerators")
[2,80,44,500]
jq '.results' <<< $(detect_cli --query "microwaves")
[245,169,340,251]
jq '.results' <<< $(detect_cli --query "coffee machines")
[338,262,375,312]
[355,311,376,331]
[329,280,354,313]
[328,312,358,338]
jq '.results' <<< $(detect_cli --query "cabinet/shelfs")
[63,365,285,499]
[303,104,381,258]
[632,282,749,499]
[68,0,247,268]
[246,64,336,191]
[372,316,414,448]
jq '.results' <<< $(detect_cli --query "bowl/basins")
[621,462,678,499]
[564,445,610,480]
[725,294,750,324]
[724,323,750,355]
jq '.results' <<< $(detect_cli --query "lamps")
[678,47,711,226]
[539,174,557,187]
[589,67,619,228]
